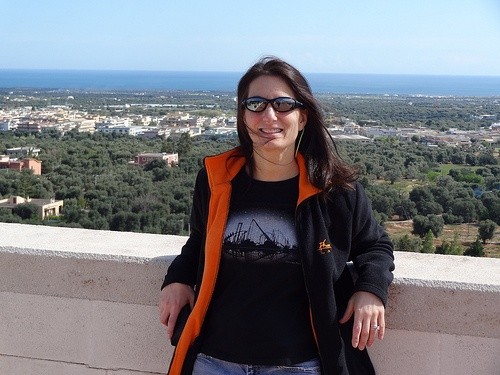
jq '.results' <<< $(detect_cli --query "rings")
[371,324,379,330]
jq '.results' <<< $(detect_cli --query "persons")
[161,55,395,375]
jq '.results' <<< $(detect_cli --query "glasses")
[241,97,306,113]
